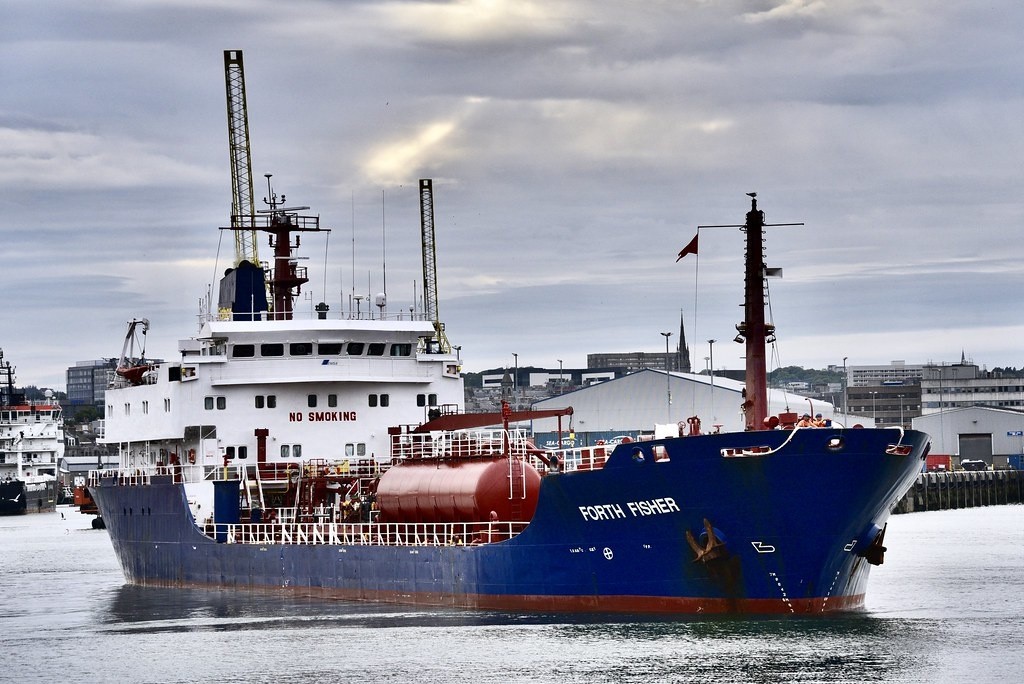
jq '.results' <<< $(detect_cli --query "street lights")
[897,394,905,427]
[557,360,563,395]
[869,391,878,428]
[660,332,673,425]
[512,353,518,390]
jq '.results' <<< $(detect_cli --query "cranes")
[223,47,272,302]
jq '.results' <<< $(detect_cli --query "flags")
[676,234,698,262]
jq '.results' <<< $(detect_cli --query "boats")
[83,172,935,619]
[0,346,65,517]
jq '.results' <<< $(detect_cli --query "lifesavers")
[188,449,195,463]
[325,463,341,475]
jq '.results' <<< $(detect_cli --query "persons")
[797,413,826,427]
[354,498,361,511]
[268,512,276,523]
[372,499,378,511]
